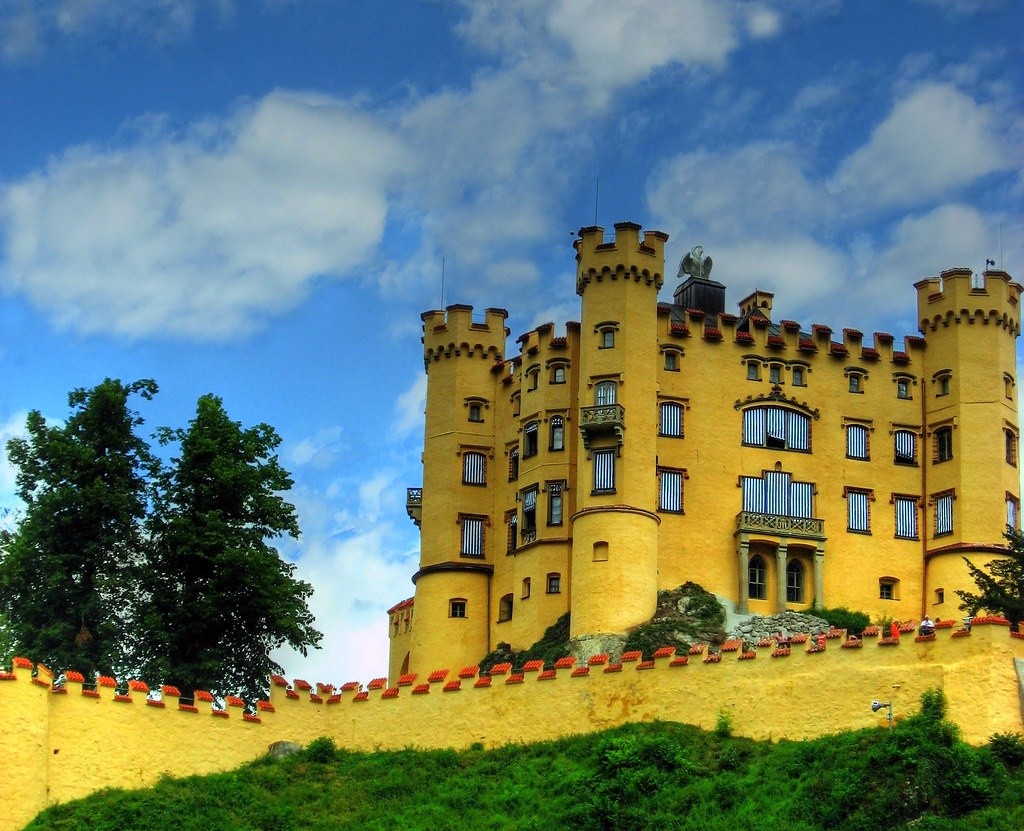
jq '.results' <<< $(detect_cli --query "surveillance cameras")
[871,700,884,712]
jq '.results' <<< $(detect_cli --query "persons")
[775,631,787,649]
[921,615,941,628]
[817,628,826,636]
